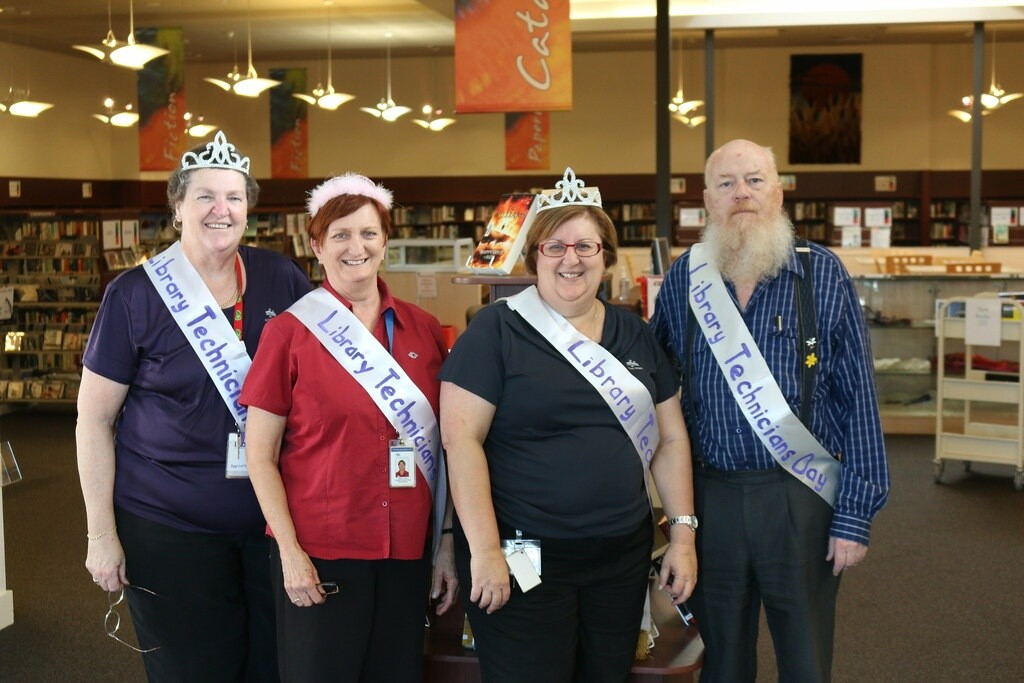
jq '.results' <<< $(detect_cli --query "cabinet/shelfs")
[0,169,1024,484]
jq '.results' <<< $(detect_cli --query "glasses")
[103,582,169,652]
[536,239,602,257]
[316,581,340,598]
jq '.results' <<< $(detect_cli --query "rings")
[294,598,299,602]
[92,578,99,583]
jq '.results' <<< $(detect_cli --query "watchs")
[668,514,698,530]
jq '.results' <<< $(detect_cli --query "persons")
[396,459,410,477]
[647,138,889,682]
[435,168,699,682]
[236,174,458,683]
[75,130,311,683]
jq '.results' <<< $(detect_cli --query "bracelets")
[442,528,451,535]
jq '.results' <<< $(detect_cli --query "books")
[989,205,1024,244]
[466,194,537,277]
[796,202,906,249]
[622,203,656,241]
[1,213,311,399]
[393,206,489,242]
[932,203,956,239]
[935,291,1024,336]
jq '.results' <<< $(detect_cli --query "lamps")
[0,0,456,138]
[947,28,1024,124]
[667,32,706,129]
